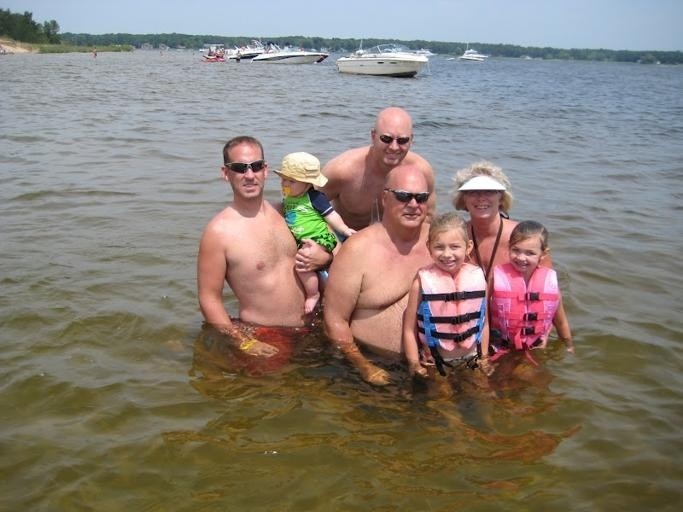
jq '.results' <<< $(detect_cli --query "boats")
[335,40,429,78]
[446,43,487,61]
[198,38,438,65]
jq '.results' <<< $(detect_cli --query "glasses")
[374,131,411,146]
[386,189,428,204]
[224,160,264,174]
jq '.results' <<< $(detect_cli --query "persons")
[93,47,97,56]
[207,39,291,59]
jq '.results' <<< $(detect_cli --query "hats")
[273,151,328,188]
[457,174,505,193]
